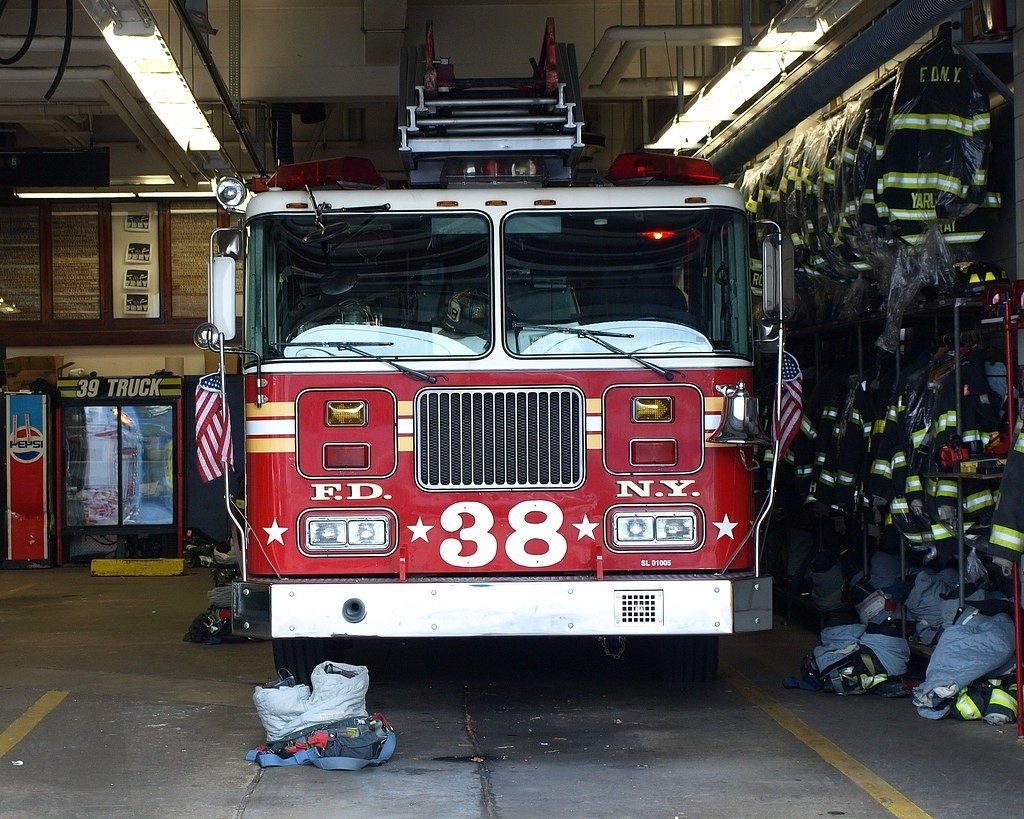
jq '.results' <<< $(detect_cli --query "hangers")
[930,346,1004,381]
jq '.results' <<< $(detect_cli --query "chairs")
[579,286,688,312]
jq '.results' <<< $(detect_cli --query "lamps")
[13,184,215,198]
[102,19,221,151]
[644,16,830,149]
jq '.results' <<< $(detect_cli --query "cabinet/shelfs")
[899,300,1016,662]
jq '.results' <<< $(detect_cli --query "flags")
[195,370,235,483]
[771,351,803,460]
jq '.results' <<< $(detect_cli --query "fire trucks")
[193,19,799,687]
